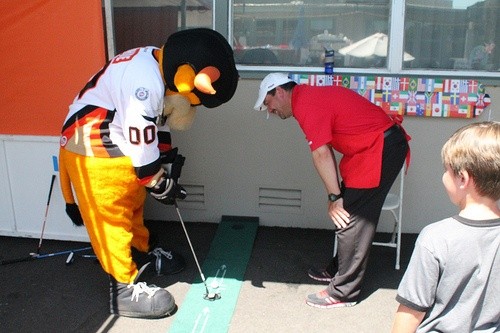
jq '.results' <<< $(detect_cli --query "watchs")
[329,193,343,202]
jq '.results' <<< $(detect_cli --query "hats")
[254,72,293,112]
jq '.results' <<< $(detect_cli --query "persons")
[253,72,411,307]
[392,121,500,333]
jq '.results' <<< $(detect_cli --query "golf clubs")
[65,251,97,265]
[25,173,56,259]
[28,247,92,260]
[172,200,223,300]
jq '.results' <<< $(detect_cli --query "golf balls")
[209,279,219,290]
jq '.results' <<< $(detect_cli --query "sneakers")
[306,289,356,309]
[307,268,332,281]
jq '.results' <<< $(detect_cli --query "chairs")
[334,158,405,269]
[454,45,488,70]
[243,48,278,65]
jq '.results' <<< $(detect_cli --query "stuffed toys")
[59,28,239,317]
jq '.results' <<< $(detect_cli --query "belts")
[383,126,398,138]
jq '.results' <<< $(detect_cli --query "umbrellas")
[339,33,415,61]
